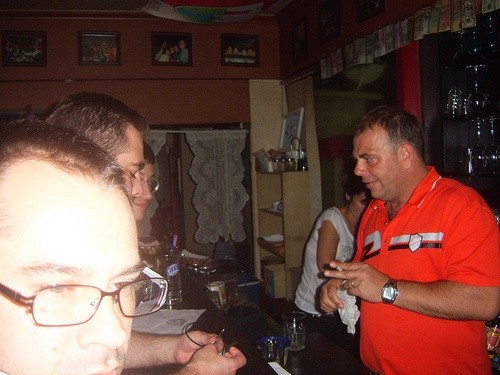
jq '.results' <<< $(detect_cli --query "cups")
[160,255,182,307]
[281,311,307,355]
[255,334,291,367]
[206,280,226,308]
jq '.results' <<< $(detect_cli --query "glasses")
[0,267,171,327]
[139,176,160,194]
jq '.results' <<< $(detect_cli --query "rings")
[350,280,354,287]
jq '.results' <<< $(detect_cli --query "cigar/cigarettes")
[336,265,343,271]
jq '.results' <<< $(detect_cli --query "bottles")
[273,158,308,172]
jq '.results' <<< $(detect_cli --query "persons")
[0,114,168,375]
[6,39,40,62]
[294,164,372,361]
[225,46,255,57]
[320,105,500,375]
[42,92,246,375]
[155,40,188,62]
[131,141,156,221]
[90,45,116,61]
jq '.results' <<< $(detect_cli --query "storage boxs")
[238,273,261,301]
[264,264,285,299]
[236,297,272,326]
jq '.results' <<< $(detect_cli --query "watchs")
[381,278,397,304]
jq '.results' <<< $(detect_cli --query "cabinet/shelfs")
[256,171,312,302]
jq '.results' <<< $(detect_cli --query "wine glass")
[446,14,500,177]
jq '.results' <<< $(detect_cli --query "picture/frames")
[221,32,261,68]
[278,108,305,149]
[77,30,122,66]
[1,30,48,67]
[150,32,192,66]
